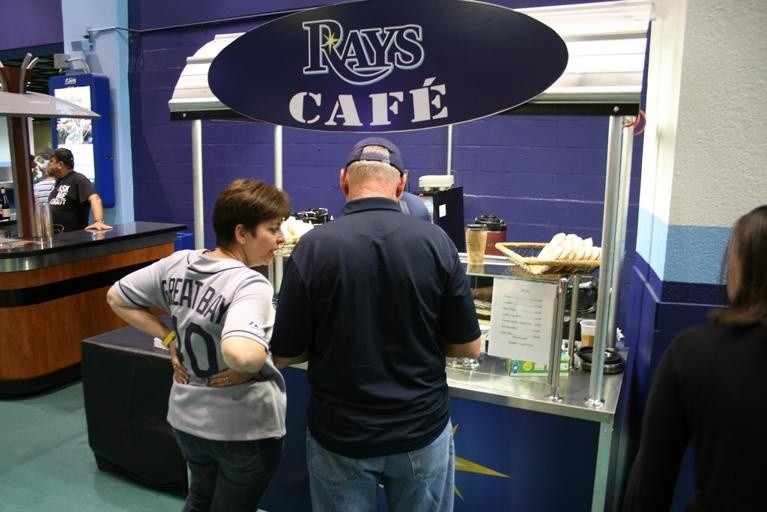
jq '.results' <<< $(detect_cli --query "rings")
[223,376,230,382]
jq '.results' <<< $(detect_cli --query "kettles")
[32,201,56,238]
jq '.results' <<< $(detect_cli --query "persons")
[105,178,288,511]
[612,205,766,511]
[45,147,112,234]
[267,137,482,511]
[27,147,56,213]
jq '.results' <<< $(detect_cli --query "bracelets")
[161,329,177,350]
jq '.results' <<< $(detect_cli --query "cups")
[579,320,596,348]
[465,224,488,266]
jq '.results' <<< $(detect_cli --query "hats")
[344,137,404,175]
[39,148,74,167]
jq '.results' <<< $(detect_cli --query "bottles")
[0,187,12,221]
